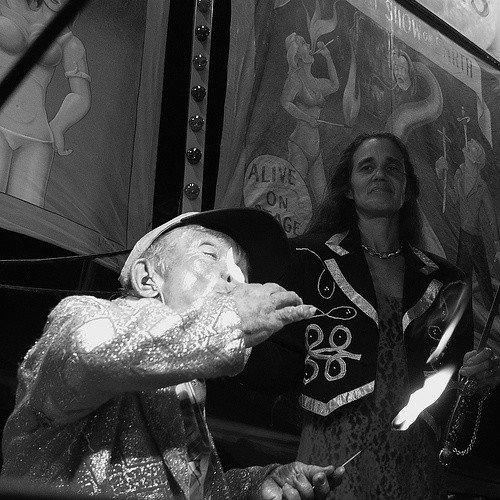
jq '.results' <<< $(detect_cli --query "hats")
[117,206,294,295]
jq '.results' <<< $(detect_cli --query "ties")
[175,382,207,500]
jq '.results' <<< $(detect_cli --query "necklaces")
[361,242,404,260]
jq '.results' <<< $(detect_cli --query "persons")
[287,134,500,500]
[434,139,500,310]
[382,50,444,145]
[279,32,340,207]
[0,0,92,209]
[1,206,348,500]
